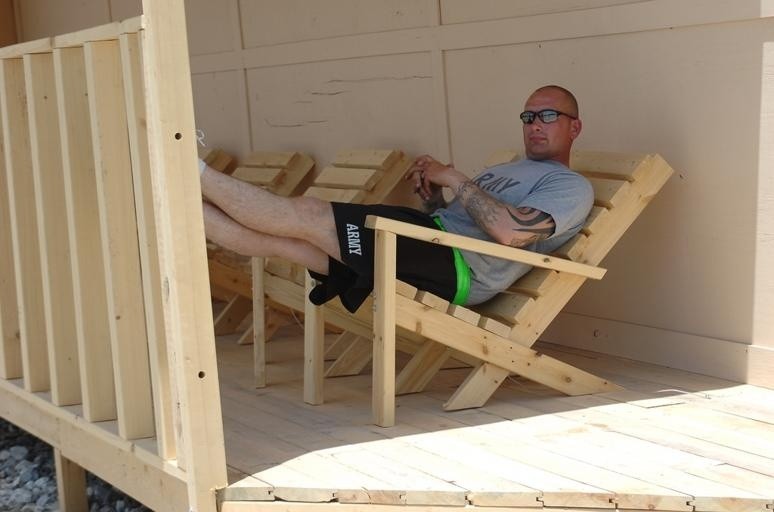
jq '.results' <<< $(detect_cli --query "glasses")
[519,109,576,125]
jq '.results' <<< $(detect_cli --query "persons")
[198,85,596,315]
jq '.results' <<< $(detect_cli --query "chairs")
[187,150,674,428]
